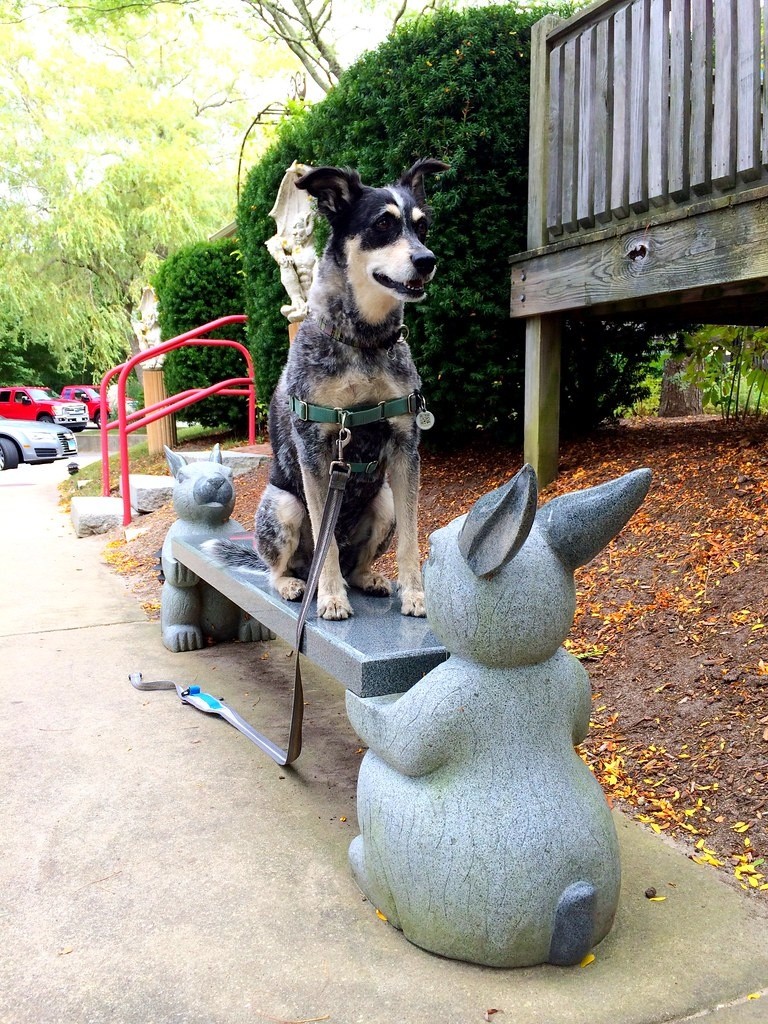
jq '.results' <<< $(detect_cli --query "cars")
[0,415,79,472]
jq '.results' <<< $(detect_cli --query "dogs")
[199,156,453,621]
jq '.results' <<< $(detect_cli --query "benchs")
[173,530,451,699]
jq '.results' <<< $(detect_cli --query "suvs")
[61,385,138,430]
[0,387,89,433]
